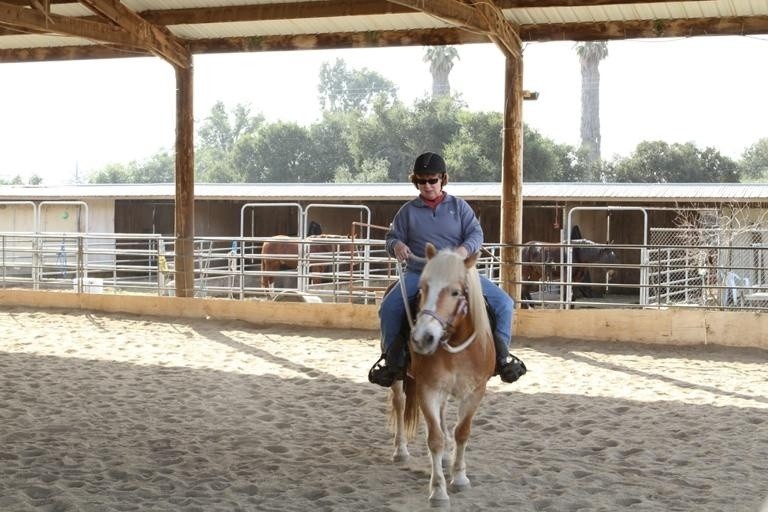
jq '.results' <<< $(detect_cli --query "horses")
[380,242,497,508]
[522,239,616,309]
[261,233,361,300]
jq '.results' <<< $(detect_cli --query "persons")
[375,152,521,386]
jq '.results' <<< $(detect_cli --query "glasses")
[418,178,437,184]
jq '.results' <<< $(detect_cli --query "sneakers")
[500,363,520,377]
[374,365,407,380]
